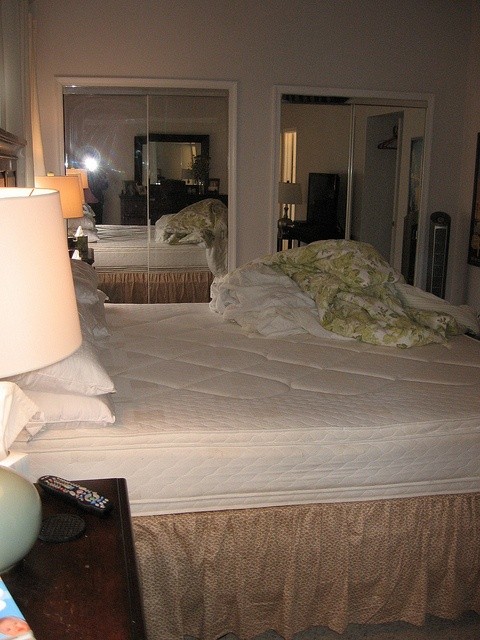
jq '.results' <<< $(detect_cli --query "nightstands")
[2,477,151,639]
[69,247,94,264]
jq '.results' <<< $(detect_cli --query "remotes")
[38,472,112,517]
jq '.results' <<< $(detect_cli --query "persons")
[83,168,105,225]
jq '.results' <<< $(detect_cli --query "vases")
[198,180,205,195]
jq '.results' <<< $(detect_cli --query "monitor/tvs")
[304,172,341,234]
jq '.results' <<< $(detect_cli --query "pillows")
[17,384,116,437]
[69,213,95,230]
[66,167,88,189]
[83,289,109,336]
[75,229,100,242]
[67,173,86,205]
[0,304,115,398]
[70,257,99,308]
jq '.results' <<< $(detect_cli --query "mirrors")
[269,84,434,289]
[55,77,240,302]
[134,135,209,186]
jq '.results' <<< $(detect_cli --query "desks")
[278,233,300,253]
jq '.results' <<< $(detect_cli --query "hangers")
[378,126,397,151]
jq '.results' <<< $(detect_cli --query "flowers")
[188,155,211,182]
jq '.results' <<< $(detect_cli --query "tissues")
[0,380,45,481]
[74,225,88,252]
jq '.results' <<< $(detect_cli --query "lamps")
[180,168,193,183]
[278,180,303,235]
[2,186,84,575]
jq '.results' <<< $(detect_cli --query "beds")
[72,224,228,303]
[2,128,480,639]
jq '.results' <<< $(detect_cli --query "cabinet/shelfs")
[118,194,154,224]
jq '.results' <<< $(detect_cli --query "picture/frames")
[467,131,479,268]
[407,136,423,215]
